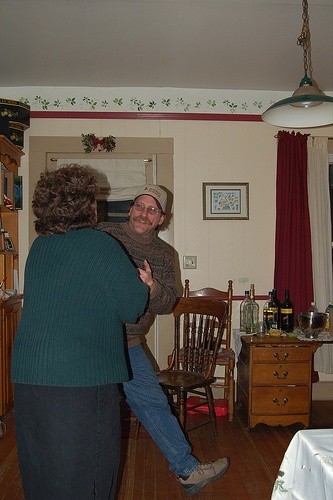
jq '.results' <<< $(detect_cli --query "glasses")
[134,202,160,215]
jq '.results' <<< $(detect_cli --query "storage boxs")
[0,99,30,150]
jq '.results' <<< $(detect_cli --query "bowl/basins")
[297,312,327,339]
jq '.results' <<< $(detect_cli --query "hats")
[133,184,167,213]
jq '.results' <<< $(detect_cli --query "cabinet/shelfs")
[0,134,23,418]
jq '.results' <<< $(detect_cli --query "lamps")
[260,0,333,129]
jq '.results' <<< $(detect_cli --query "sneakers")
[176,457,229,496]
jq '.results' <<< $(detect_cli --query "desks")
[271,428,333,500]
[232,329,333,432]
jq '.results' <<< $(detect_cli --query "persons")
[8,163,150,500]
[97,185,229,496]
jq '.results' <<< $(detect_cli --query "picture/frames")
[4,176,22,210]
[203,182,249,220]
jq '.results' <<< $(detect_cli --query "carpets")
[119,398,141,440]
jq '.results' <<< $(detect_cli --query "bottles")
[246,284,259,334]
[307,302,318,312]
[280,290,294,333]
[325,302,333,332]
[240,291,253,332]
[263,292,279,335]
[269,289,279,330]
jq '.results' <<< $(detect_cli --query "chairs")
[131,280,236,440]
